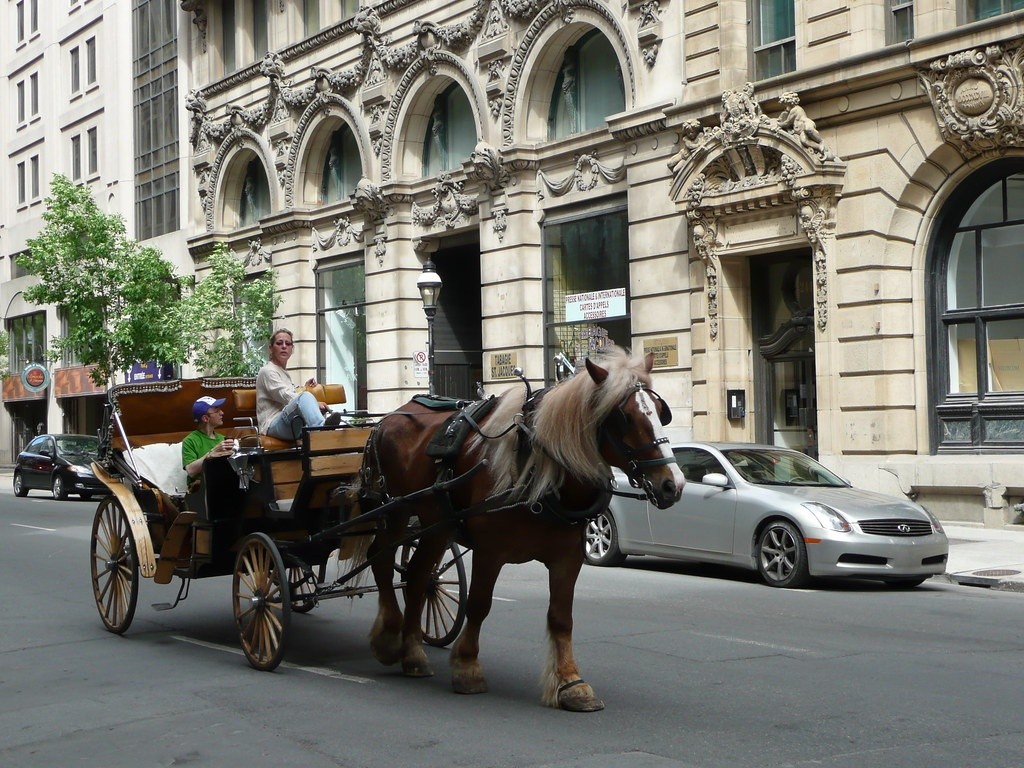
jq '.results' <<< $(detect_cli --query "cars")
[13,434,113,500]
[585,442,949,589]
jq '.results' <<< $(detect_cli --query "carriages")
[89,342,685,713]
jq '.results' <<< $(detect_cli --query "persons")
[666,118,703,178]
[256,328,340,441]
[776,92,824,154]
[182,396,234,495]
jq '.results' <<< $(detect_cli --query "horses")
[340,344,686,712]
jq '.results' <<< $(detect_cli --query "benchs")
[96,376,346,556]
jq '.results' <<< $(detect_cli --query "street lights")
[416,258,442,395]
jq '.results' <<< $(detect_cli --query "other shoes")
[319,412,341,431]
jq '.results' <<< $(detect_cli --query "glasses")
[208,408,222,414]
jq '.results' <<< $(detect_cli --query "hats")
[192,395,226,422]
